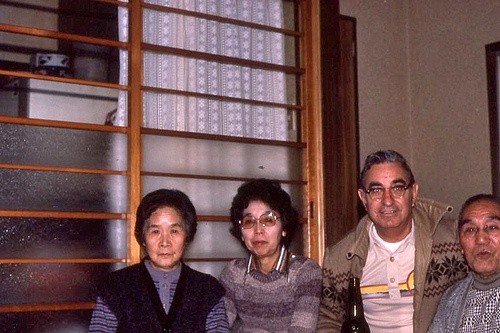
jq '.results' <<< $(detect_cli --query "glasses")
[233,210,286,230]
[362,183,413,201]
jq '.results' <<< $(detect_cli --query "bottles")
[340,277,370,333]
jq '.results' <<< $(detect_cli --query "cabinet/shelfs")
[0,0,324,333]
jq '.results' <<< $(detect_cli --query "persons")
[427,194,500,333]
[88,188,231,333]
[316,149,472,333]
[218,177,323,333]
